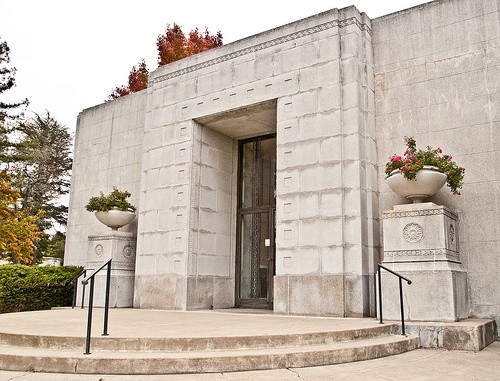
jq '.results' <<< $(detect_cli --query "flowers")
[85,186,137,212]
[385,135,466,196]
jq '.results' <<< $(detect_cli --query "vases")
[94,206,136,237]
[386,165,448,210]
